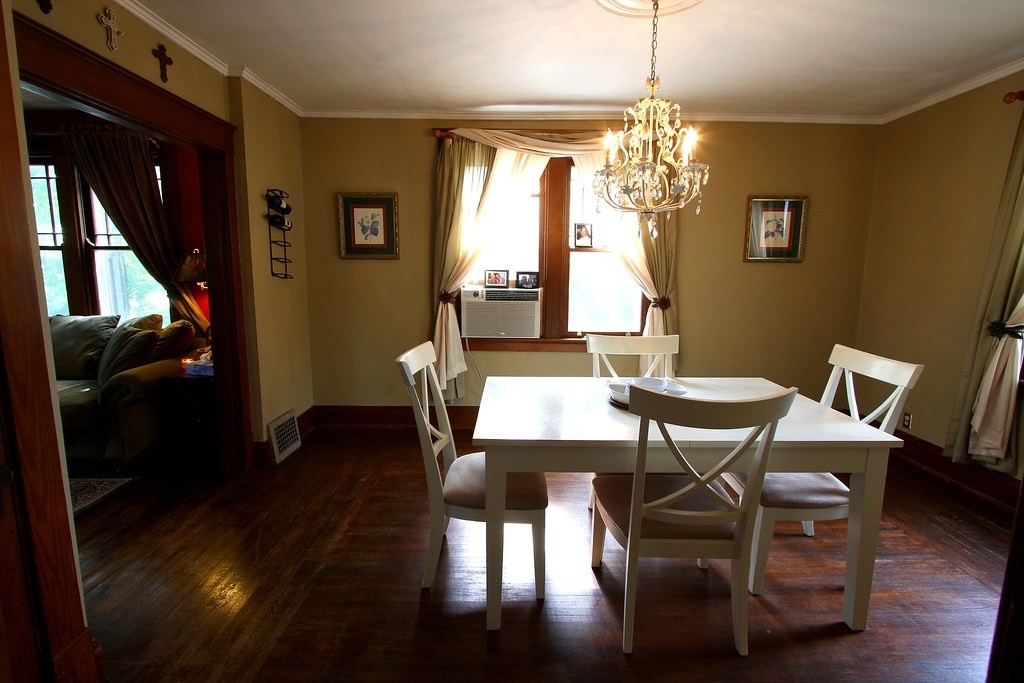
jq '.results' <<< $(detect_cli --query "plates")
[608,393,630,407]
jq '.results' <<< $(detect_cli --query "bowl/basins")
[608,377,687,404]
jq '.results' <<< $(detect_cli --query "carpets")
[68,473,143,516]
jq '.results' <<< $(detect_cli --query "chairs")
[589,383,798,655]
[587,333,680,512]
[395,341,548,602]
[697,343,926,596]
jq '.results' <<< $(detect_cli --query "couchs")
[50,317,207,469]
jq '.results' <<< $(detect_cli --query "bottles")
[576,330,583,337]
[266,213,292,230]
[266,193,290,215]
[626,331,631,336]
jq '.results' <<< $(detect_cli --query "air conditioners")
[460,285,544,340]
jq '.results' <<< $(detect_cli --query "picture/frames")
[516,272,539,288]
[742,195,809,263]
[484,270,509,288]
[337,191,400,261]
[575,223,592,247]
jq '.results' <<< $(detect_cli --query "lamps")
[592,0,710,237]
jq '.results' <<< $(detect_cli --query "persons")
[491,273,504,284]
[520,276,527,286]
[579,227,591,246]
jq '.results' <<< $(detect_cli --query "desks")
[472,377,903,631]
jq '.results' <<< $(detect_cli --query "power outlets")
[903,413,912,430]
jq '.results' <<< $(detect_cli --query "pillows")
[48,313,196,387]
